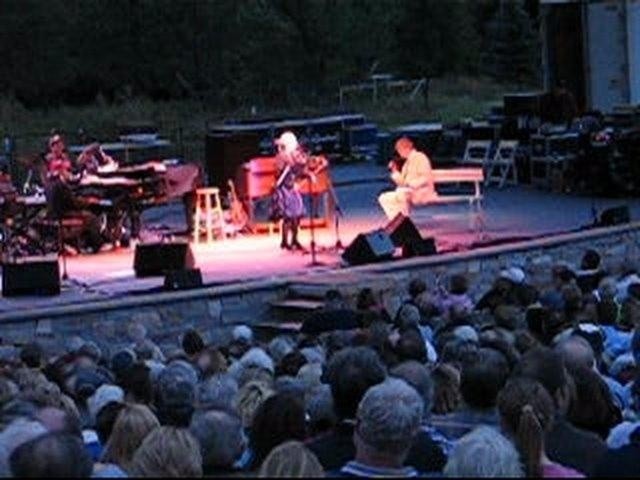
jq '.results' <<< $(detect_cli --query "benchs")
[429,167,486,232]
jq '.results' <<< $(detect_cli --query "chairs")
[456,139,520,191]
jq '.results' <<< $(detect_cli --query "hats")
[500,267,525,284]
[232,325,253,343]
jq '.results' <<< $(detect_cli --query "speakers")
[385,211,424,246]
[164,267,203,290]
[2,257,60,296]
[133,242,196,277]
[597,204,630,225]
[206,132,260,210]
[402,237,438,257]
[341,226,397,265]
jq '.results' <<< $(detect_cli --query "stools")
[194,187,228,245]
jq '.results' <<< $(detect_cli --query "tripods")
[57,219,88,288]
[324,209,348,251]
[300,177,330,269]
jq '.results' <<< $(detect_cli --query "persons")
[1,132,144,261]
[496,78,640,201]
[2,249,639,479]
[377,137,436,221]
[273,130,319,254]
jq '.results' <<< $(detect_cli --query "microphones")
[389,156,400,171]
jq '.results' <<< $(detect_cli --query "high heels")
[281,240,304,251]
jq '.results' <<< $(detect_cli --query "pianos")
[65,161,205,246]
